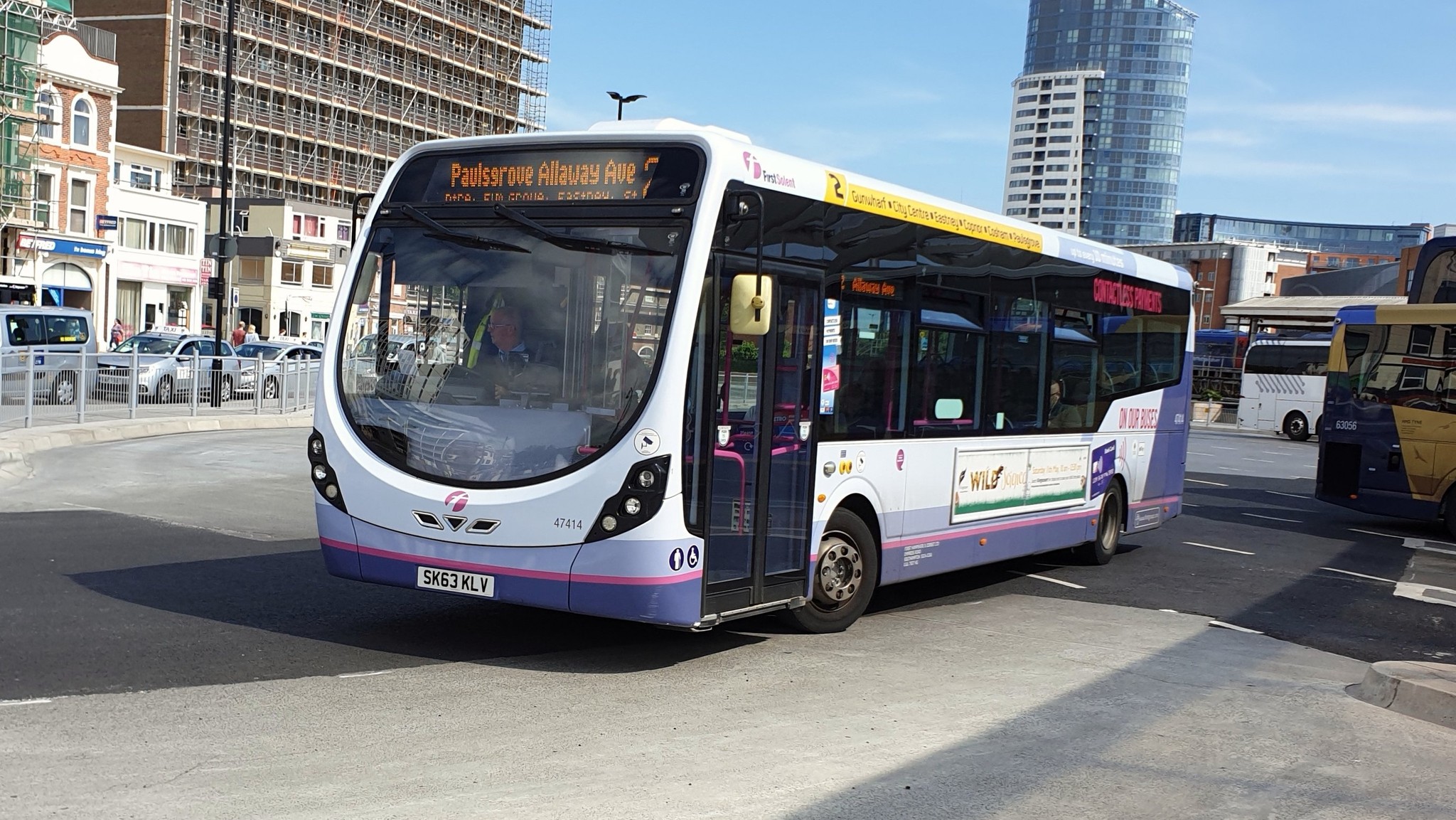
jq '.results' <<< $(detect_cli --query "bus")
[1236,340,1332,441]
[1315,302,1456,526]
[307,116,1195,635]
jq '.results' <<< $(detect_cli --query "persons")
[278,329,286,338]
[244,325,260,352]
[108,318,124,352]
[415,340,447,366]
[1027,380,1081,429]
[231,321,248,350]
[471,305,559,401]
[301,331,307,340]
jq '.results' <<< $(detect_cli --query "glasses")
[488,322,516,331]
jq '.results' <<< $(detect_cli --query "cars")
[97,326,241,401]
[233,336,324,400]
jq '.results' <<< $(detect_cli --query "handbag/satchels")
[116,334,123,342]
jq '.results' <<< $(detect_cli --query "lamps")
[265,313,268,319]
[274,314,277,319]
[336,245,347,252]
[305,316,307,322]
[276,242,281,247]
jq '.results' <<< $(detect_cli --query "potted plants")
[1192,388,1223,422]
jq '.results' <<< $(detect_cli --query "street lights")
[285,293,313,337]
[607,89,647,121]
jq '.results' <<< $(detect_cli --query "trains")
[1192,329,1331,400]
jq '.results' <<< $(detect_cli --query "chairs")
[862,357,1158,438]
[13,318,29,343]
[47,321,68,343]
[202,345,211,355]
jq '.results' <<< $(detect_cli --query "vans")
[0,303,98,406]
[349,334,456,395]
